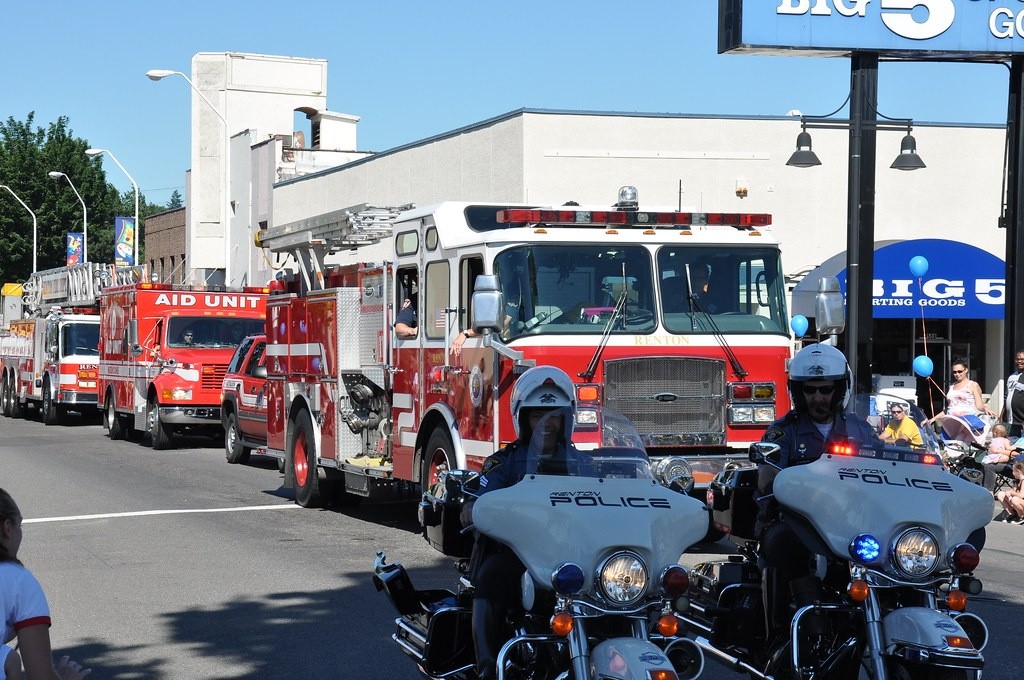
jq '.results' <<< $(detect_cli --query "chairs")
[649,277,686,312]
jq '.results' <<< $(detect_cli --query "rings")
[455,347,459,349]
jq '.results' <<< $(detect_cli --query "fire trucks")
[97,285,271,451]
[252,176,847,509]
[0,262,152,426]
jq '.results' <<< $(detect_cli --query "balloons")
[913,355,933,377]
[791,315,808,337]
[909,256,929,277]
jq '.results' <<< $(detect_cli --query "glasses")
[891,411,902,414]
[800,384,836,396]
[953,368,967,373]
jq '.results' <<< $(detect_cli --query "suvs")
[219,333,287,472]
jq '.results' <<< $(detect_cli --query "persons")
[460,366,604,680]
[0,486,92,680]
[183,330,194,345]
[62,326,88,355]
[394,291,419,340]
[293,132,304,149]
[929,350,1024,525]
[879,404,924,450]
[230,321,243,343]
[667,259,726,313]
[450,268,530,357]
[754,343,874,673]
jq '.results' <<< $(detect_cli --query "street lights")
[85,147,140,266]
[48,171,88,263]
[145,68,232,286]
[0,185,36,300]
[786,53,929,423]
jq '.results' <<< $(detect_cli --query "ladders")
[258,202,416,240]
[28,262,144,307]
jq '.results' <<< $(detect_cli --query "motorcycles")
[370,400,714,680]
[683,394,994,680]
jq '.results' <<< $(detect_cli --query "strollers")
[929,415,989,487]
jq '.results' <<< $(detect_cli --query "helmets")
[789,344,853,411]
[510,366,578,444]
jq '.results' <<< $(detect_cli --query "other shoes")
[1001,515,1017,523]
[1011,516,1024,525]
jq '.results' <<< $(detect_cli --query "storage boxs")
[371,551,468,680]
[692,556,761,649]
[707,465,760,541]
[418,469,480,558]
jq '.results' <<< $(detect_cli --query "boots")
[471,597,497,680]
[760,568,792,676]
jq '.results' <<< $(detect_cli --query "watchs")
[463,330,470,338]
